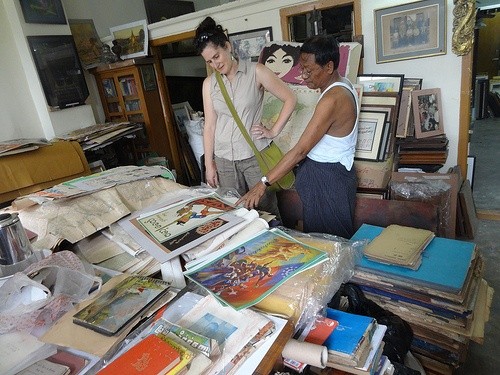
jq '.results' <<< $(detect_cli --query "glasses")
[193,31,222,48]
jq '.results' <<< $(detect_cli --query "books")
[0,195,477,375]
[95,333,182,375]
[101,74,141,113]
[183,228,327,312]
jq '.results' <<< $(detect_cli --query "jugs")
[0,212,38,275]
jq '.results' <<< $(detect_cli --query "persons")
[234,34,360,240]
[419,98,441,133]
[392,19,430,49]
[192,15,299,230]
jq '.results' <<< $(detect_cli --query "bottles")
[101,44,114,61]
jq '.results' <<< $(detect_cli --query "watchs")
[261,175,271,187]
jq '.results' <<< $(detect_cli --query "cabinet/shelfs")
[95,65,175,171]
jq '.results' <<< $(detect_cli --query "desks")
[393,138,450,173]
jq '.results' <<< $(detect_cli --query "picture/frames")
[354,74,445,160]
[109,19,149,60]
[69,18,108,70]
[26,34,92,111]
[19,0,68,24]
[140,65,158,92]
[373,0,448,64]
[227,27,272,59]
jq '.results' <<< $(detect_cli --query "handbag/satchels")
[0,250,102,335]
[257,141,296,192]
